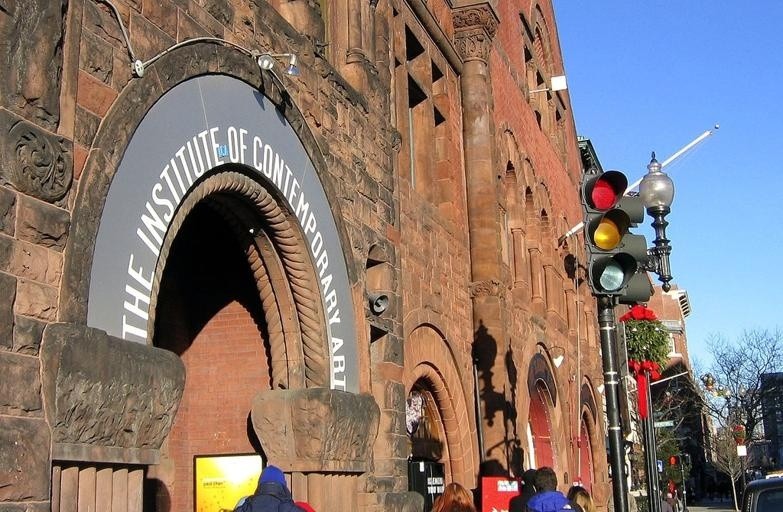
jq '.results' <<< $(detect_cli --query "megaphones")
[368,293,388,314]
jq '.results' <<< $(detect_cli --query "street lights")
[586,150,675,512]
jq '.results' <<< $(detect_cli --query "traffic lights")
[667,454,692,467]
[584,169,652,306]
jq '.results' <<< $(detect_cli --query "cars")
[740,476,783,512]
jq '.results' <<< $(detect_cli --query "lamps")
[539,346,565,369]
[589,374,604,394]
[254,52,299,77]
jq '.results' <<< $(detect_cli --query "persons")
[233,465,316,512]
[430,481,477,512]
[508,466,595,512]
